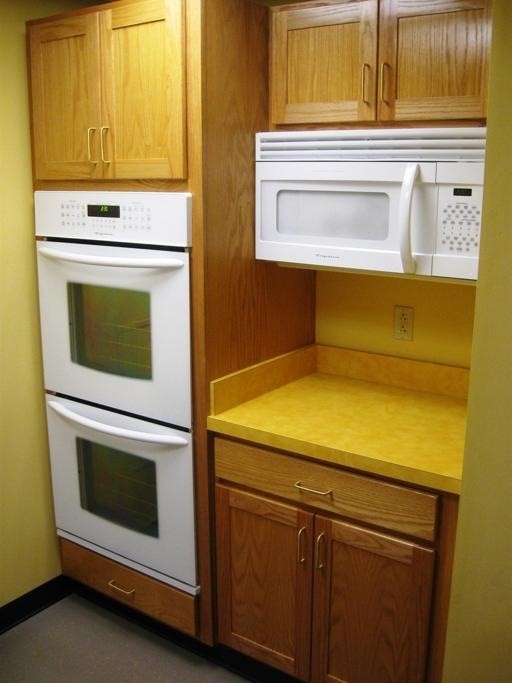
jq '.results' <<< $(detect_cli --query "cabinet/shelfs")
[208,432,460,682]
[263,2,494,131]
[26,0,266,188]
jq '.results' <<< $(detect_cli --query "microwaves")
[251,127,482,281]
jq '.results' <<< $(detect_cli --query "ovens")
[33,189,200,589]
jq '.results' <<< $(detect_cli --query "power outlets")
[392,303,417,343]
